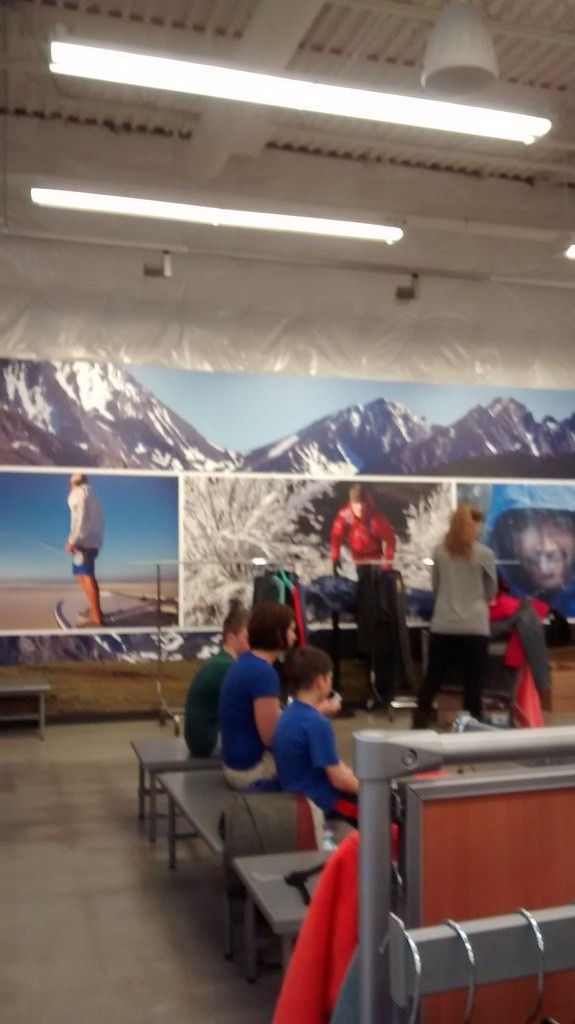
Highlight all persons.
[183,503,497,869]
[332,483,395,592]
[504,508,575,619]
[66,475,107,629]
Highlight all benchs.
[156,769,236,959]
[131,736,191,841]
[232,850,334,984]
[0,675,52,739]
